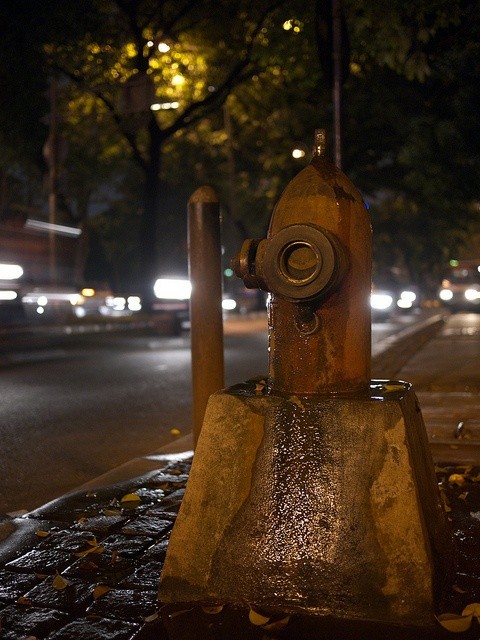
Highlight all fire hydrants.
[228,125,373,396]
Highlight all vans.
[435,265,480,314]
